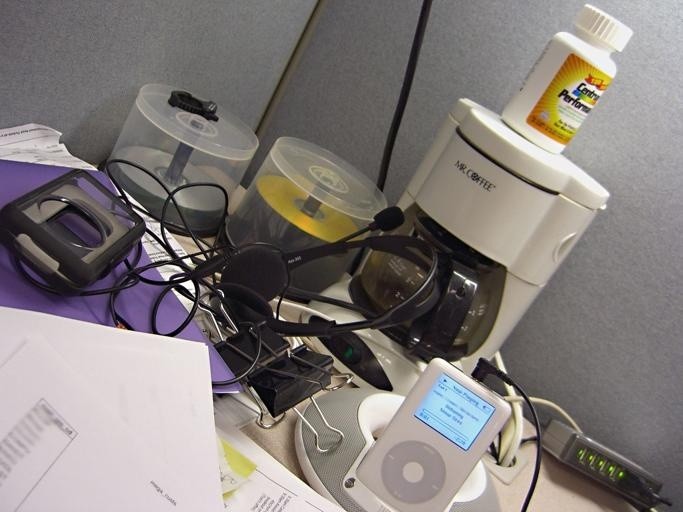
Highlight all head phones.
[212,235,442,336]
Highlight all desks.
[106,165,657,511]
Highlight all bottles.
[502,5,633,153]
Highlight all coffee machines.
[298,95,612,395]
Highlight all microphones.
[333,205,405,241]
